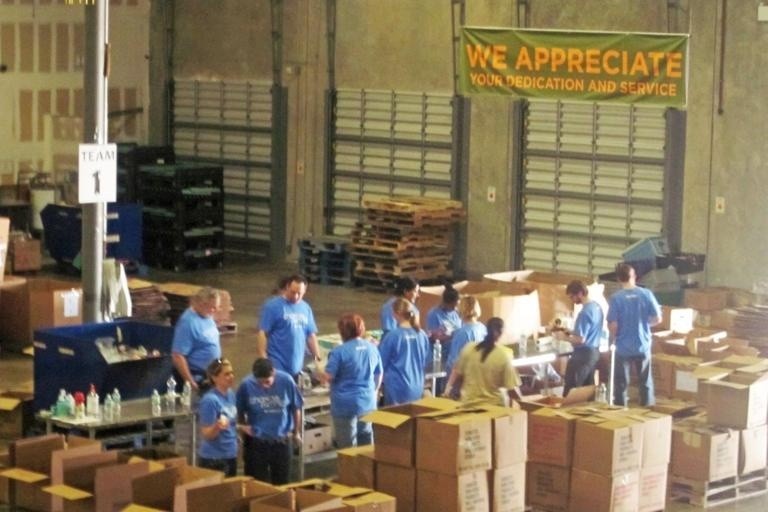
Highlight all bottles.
[152,387,162,416]
[85,383,100,419]
[57,385,85,421]
[433,339,442,361]
[166,374,177,406]
[183,382,191,406]
[598,382,607,402]
[112,388,121,415]
[105,392,113,422]
[519,330,527,348]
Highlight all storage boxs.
[0,217,83,339]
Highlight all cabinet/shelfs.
[141,161,226,273]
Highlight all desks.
[0,184,30,231]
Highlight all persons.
[427,279,461,341]
[378,275,421,338]
[379,297,434,403]
[172,287,222,393]
[315,312,381,449]
[442,317,524,410]
[257,275,321,375]
[236,358,303,484]
[563,281,604,399]
[194,357,239,477]
[607,263,662,405]
[446,293,486,379]
